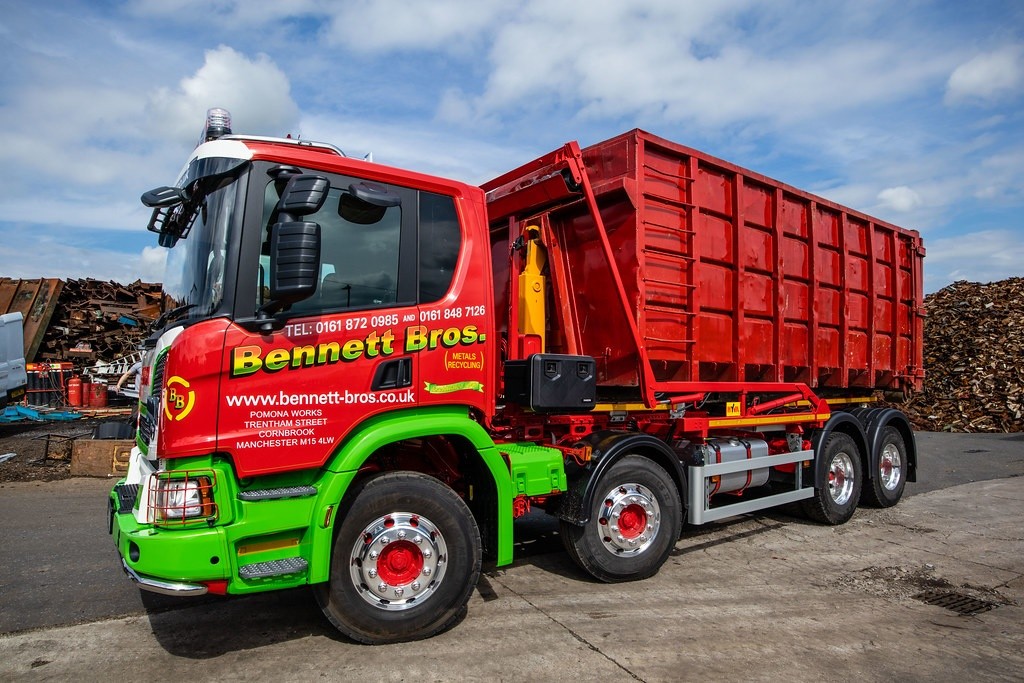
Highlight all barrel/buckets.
[69,379,90,408]
[90,382,106,407]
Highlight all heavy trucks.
[104,106,925,648]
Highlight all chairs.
[321,273,391,304]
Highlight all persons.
[115,362,142,447]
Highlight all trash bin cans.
[97,415,132,439]
